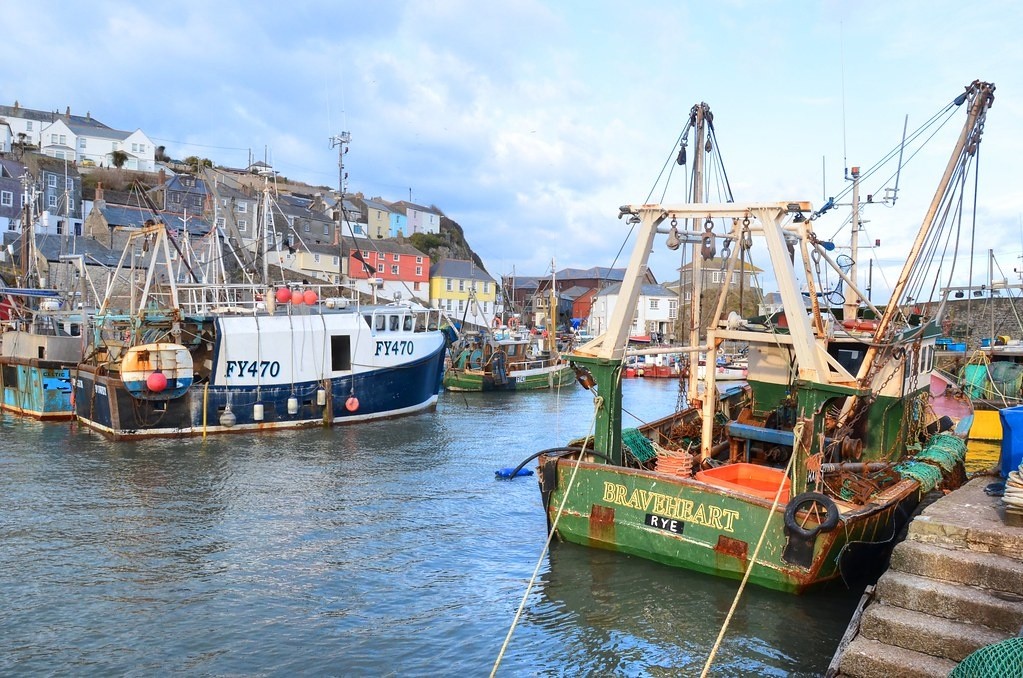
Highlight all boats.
[1,130,751,442]
[535,79,999,598]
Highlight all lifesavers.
[492,318,501,329]
[841,318,880,334]
[508,318,519,328]
[919,415,954,443]
[718,349,722,354]
[783,491,839,538]
[763,405,801,462]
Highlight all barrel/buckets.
[654,448,694,478]
